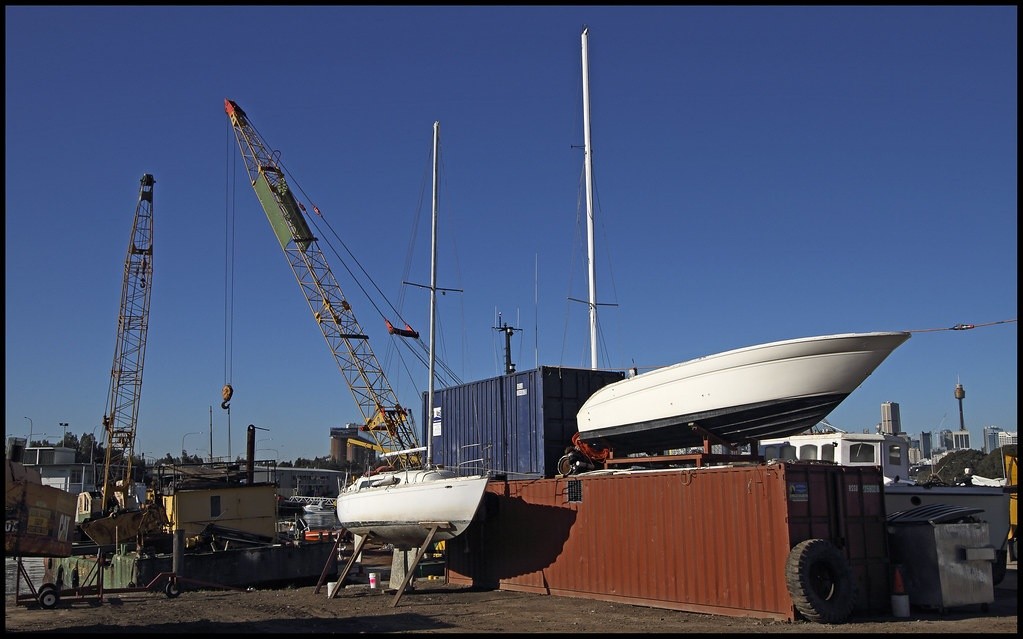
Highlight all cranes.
[81,175,168,546]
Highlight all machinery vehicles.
[220,98,463,472]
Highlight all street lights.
[24,433,46,439]
[182,432,203,449]
[24,417,33,441]
[59,422,68,447]
[256,438,273,450]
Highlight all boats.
[576,331,912,454]
[302,500,335,515]
[305,530,339,541]
[760,433,1010,587]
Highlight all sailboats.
[337,122,488,548]
